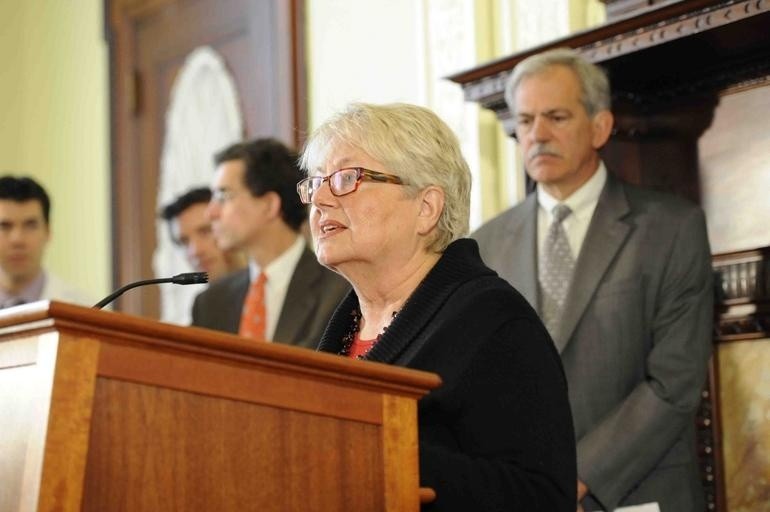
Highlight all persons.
[189,135,353,352]
[470,46,714,512]
[161,187,247,285]
[0,174,79,309]
[296,104,577,512]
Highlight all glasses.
[296,167,409,205]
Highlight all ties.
[538,204,574,333]
[238,274,267,339]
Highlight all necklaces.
[336,306,399,362]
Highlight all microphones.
[90,271,208,310]
